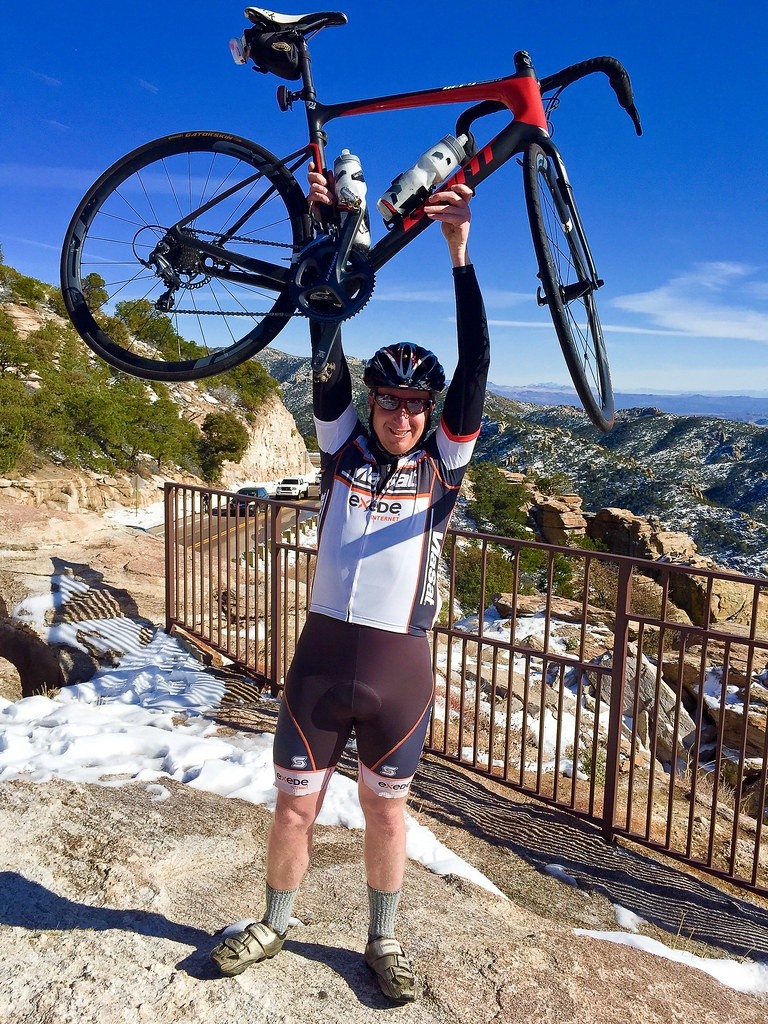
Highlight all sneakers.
[365,938,418,1003]
[210,923,289,977]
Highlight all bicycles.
[60,7,643,431]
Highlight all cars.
[230,487,271,517]
[315,471,322,485]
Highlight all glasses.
[371,391,434,415]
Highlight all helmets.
[362,342,446,392]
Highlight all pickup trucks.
[275,478,309,500]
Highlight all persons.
[204,162,491,1003]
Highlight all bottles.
[377,133,470,224]
[334,150,374,247]
